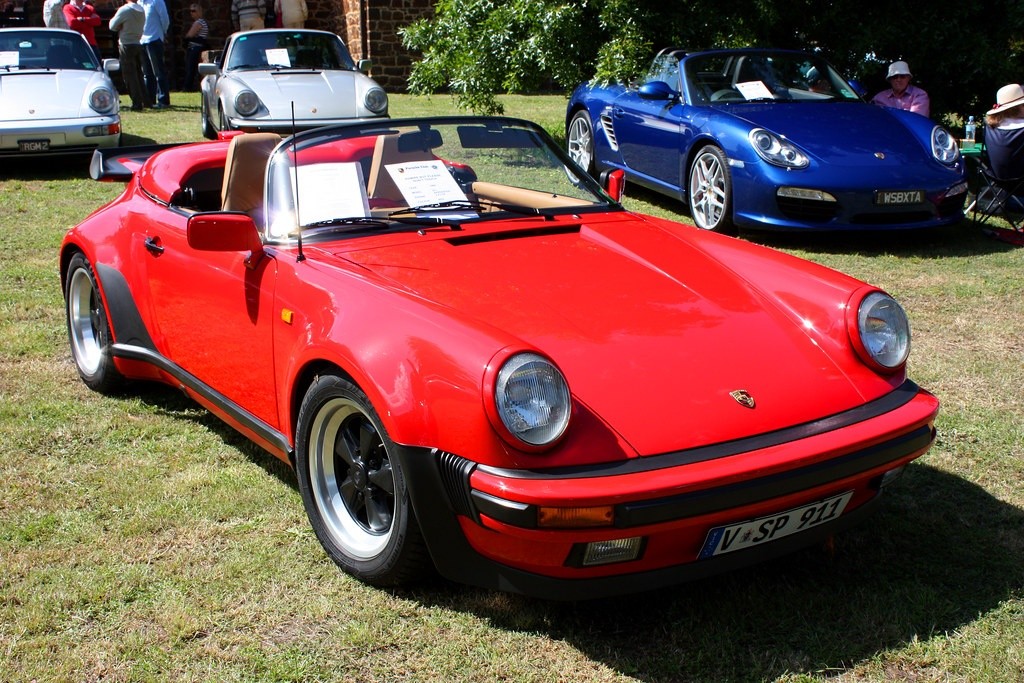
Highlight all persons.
[871,60,930,116]
[803,66,832,93]
[180,2,208,92]
[0,0,102,58]
[108,0,176,112]
[985,84,1024,192]
[232,0,266,30]
[274,0,308,28]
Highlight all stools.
[972,166,1024,237]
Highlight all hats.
[885,61,913,79]
[986,84,1024,115]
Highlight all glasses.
[189,10,196,12]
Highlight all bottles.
[966,115,975,140]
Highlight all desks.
[960,143,987,194]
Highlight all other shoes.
[152,103,169,108]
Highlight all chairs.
[719,56,739,90]
[660,51,698,92]
[221,133,293,231]
[647,47,677,79]
[731,56,774,91]
[294,49,325,68]
[234,46,264,65]
[367,135,433,202]
[46,45,72,68]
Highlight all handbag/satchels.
[276,0,283,28]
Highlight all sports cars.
[564,46,968,241]
[199,29,390,135]
[0,26,121,165]
[59,113,941,593]
